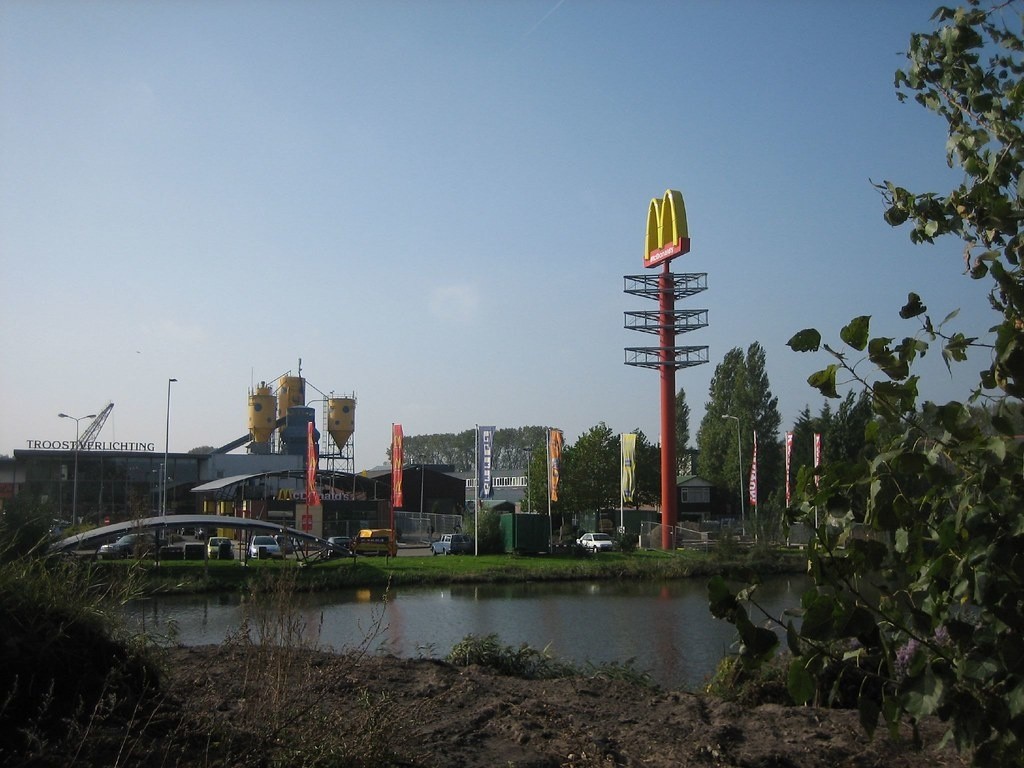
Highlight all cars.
[576,533,613,552]
[194,528,205,540]
[431,534,474,556]
[177,527,194,536]
[327,537,354,558]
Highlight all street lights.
[58,413,97,525]
[419,454,427,517]
[722,414,744,536]
[522,447,533,514]
[162,378,178,515]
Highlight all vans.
[352,528,397,556]
[207,537,232,559]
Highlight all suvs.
[100,534,155,558]
[247,535,280,558]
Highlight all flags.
[478,426,496,497]
[622,432,637,500]
[748,429,823,506]
[392,426,403,506]
[548,427,563,502]
[306,422,320,507]
